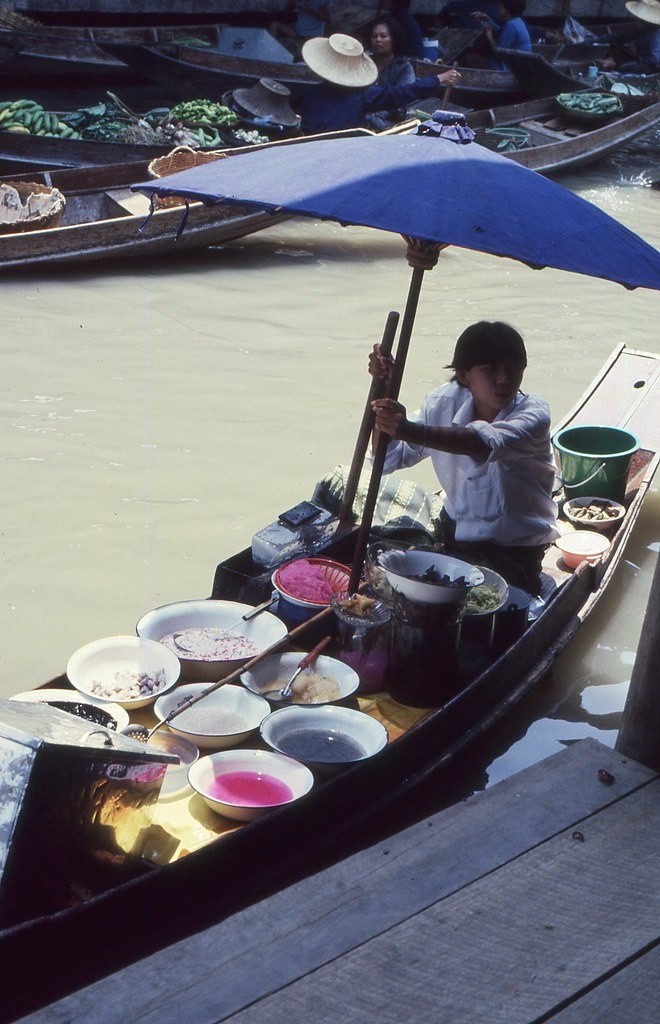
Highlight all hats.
[301,33,379,87]
[624,0,660,25]
[232,78,299,127]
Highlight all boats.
[1,0,660,284]
[0,342,660,1024]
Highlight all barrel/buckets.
[388,615,461,708]
[478,584,533,649]
[551,425,641,501]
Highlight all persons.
[368,321,556,597]
[583,0,660,74]
[268,0,557,128]
[288,34,461,133]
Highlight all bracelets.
[407,422,427,457]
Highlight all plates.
[9,689,130,734]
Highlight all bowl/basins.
[463,564,510,616]
[66,635,181,710]
[187,749,315,822]
[330,591,391,628]
[563,495,626,532]
[366,540,424,601]
[147,729,200,799]
[135,599,289,681]
[240,652,361,710]
[154,682,272,750]
[378,550,485,607]
[259,705,389,775]
[555,530,610,569]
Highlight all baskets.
[553,92,623,125]
[0,181,66,235]
[601,76,660,118]
[147,146,233,208]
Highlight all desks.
[104,189,156,219]
[520,121,582,146]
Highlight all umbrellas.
[131,110,660,596]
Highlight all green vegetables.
[463,583,502,613]
